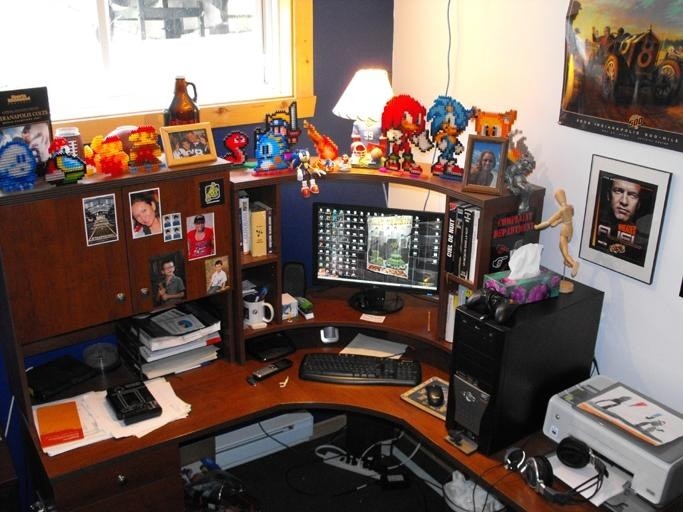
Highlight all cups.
[54,127,83,162]
[243,294,273,324]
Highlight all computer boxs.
[445,276,605,456]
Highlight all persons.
[206,260,227,295]
[187,215,213,259]
[171,131,207,161]
[533,190,582,278]
[164,213,180,242]
[154,260,184,303]
[131,197,160,236]
[606,175,637,224]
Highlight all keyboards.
[298,353,421,386]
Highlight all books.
[130,307,224,379]
[443,284,473,342]
[237,192,274,258]
[443,202,484,284]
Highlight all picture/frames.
[461,134,509,196]
[578,154,673,285]
[160,122,218,168]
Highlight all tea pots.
[169,77,200,126]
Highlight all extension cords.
[323,451,381,480]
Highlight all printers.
[542,375,683,512]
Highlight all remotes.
[252,359,292,379]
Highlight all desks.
[18,326,613,512]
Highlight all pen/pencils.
[248,287,268,302]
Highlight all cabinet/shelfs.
[1,158,242,353]
[228,156,545,364]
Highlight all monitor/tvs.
[320,202,444,315]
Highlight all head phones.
[522,436,609,506]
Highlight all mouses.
[425,384,444,406]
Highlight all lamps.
[331,68,396,142]
